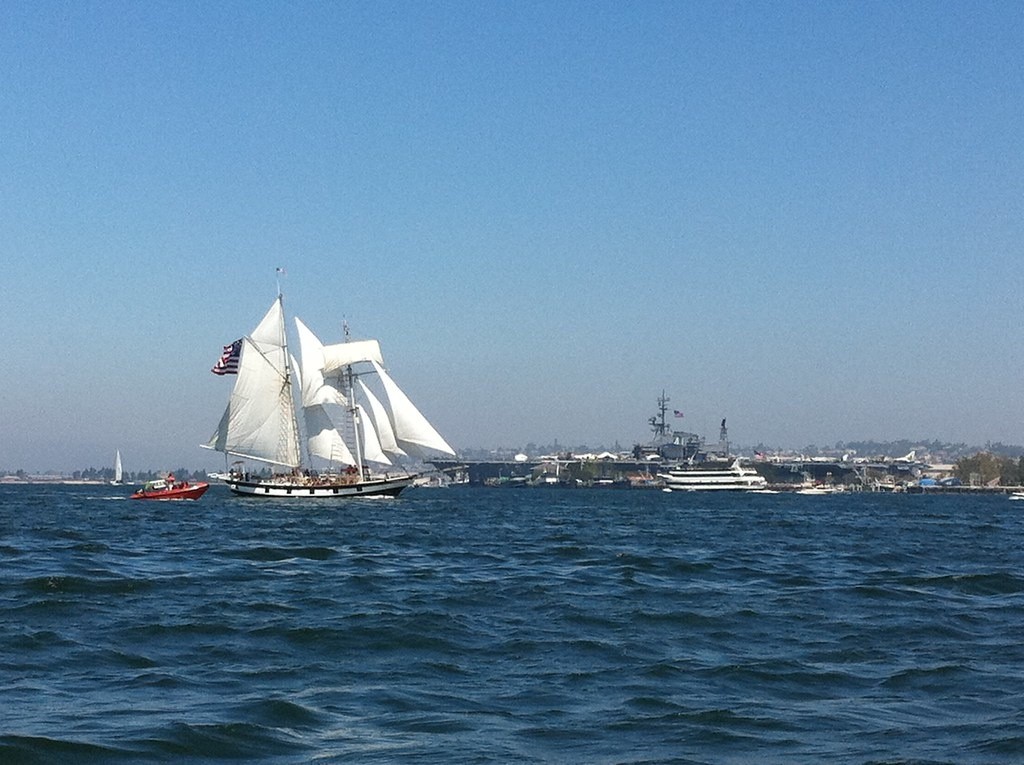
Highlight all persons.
[229,463,374,485]
[166,471,175,490]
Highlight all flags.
[211,338,243,376]
[674,410,684,417]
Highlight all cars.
[919,479,938,485]
[935,477,963,487]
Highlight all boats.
[129,470,210,501]
[144,478,167,490]
[796,488,833,495]
[657,466,769,491]
[1008,491,1024,501]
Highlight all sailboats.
[199,267,470,502]
[109,448,124,488]
